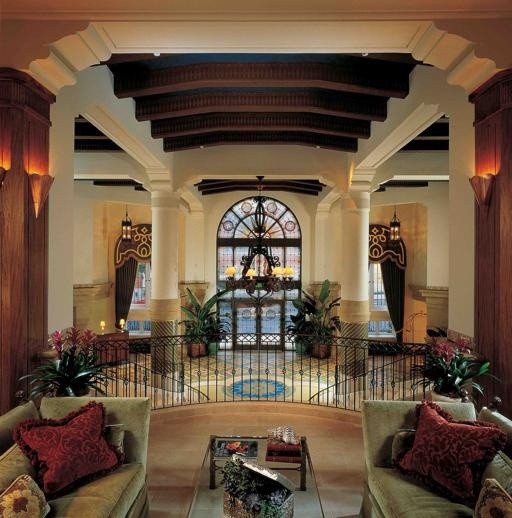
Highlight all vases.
[431,390,469,402]
[81,389,92,397]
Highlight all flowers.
[17,327,110,397]
[410,335,491,398]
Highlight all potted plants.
[285,321,312,355]
[205,312,230,355]
[292,278,341,359]
[178,286,233,358]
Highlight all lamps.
[224,175,295,306]
[390,205,401,242]
[121,203,133,241]
[119,319,126,332]
[99,320,105,335]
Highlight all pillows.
[481,449,512,497]
[12,400,123,501]
[0,442,37,495]
[391,427,417,469]
[392,400,508,511]
[105,424,126,463]
[0,474,52,517]
[472,477,512,518]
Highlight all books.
[213,440,258,461]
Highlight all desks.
[94,328,129,367]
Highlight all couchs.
[358,400,512,518]
[0,397,151,517]
